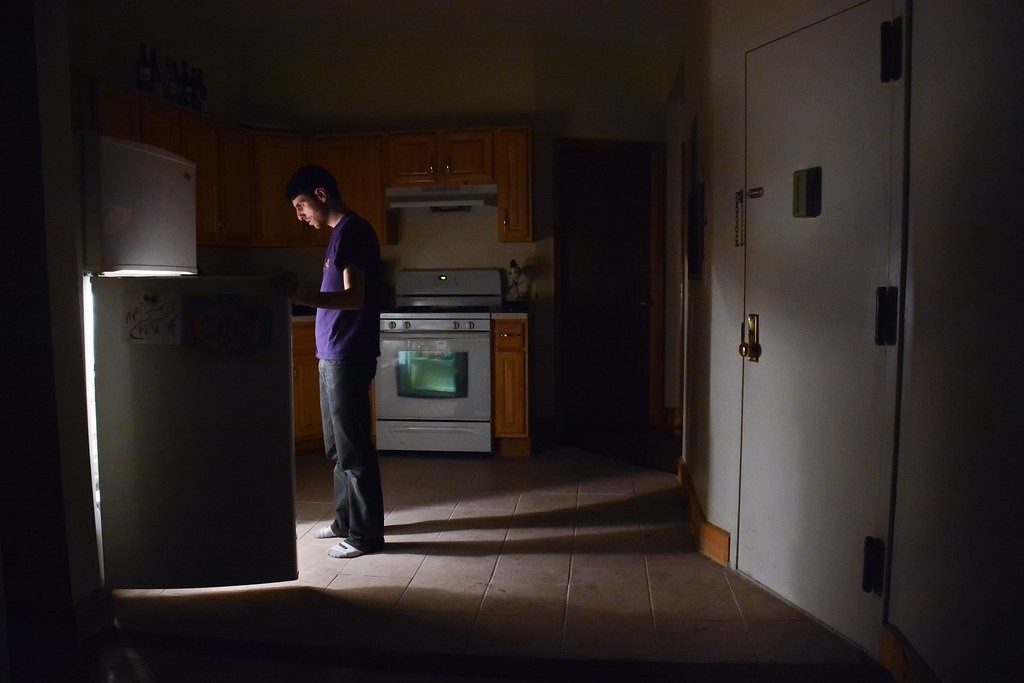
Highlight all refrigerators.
[50,133,300,599]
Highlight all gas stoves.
[380,263,505,319]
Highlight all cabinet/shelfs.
[289,308,530,458]
[69,76,536,251]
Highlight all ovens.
[376,319,490,423]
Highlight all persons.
[267,167,388,557]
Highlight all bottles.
[507,259,520,300]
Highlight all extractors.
[387,181,498,217]
